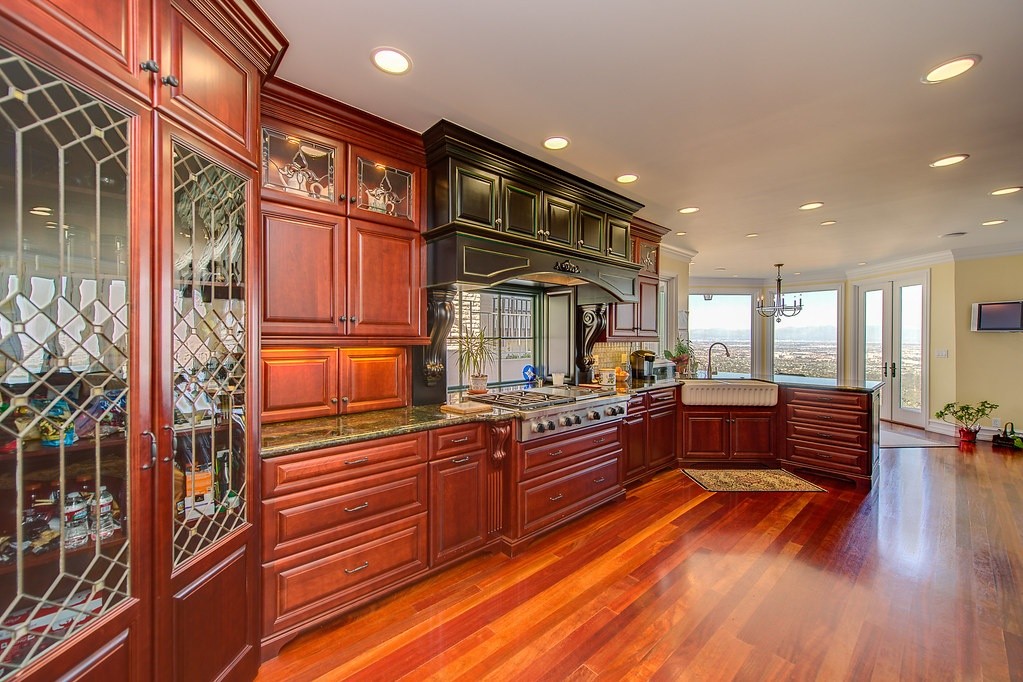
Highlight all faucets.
[707,342,730,379]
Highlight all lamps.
[756,264,805,323]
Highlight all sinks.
[679,380,723,405]
[724,380,779,406]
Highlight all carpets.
[680,468,828,493]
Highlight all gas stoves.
[466,390,577,411]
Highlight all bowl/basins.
[616,376,629,382]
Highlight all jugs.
[365,189,395,215]
[309,182,334,203]
[279,165,309,197]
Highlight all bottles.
[63,493,89,550]
[90,487,114,540]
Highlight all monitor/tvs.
[971,301,1023,333]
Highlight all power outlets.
[593,355,599,367]
[621,354,628,364]
[991,418,1002,428]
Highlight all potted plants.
[664,337,695,372]
[935,400,999,442]
[448,323,500,395]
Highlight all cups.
[599,369,616,386]
[551,372,565,386]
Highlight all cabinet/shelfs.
[577,176,645,262]
[647,387,679,478]
[499,143,582,250]
[261,345,413,424]
[0,0,290,682]
[622,391,647,489]
[780,387,882,490]
[422,118,501,231]
[595,216,672,343]
[502,418,627,559]
[0,418,244,616]
[259,76,428,232]
[260,431,430,663]
[260,200,432,346]
[428,422,502,578]
[682,411,778,470]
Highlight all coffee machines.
[630,350,657,380]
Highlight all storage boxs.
[184,492,215,520]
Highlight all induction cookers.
[522,388,617,401]
